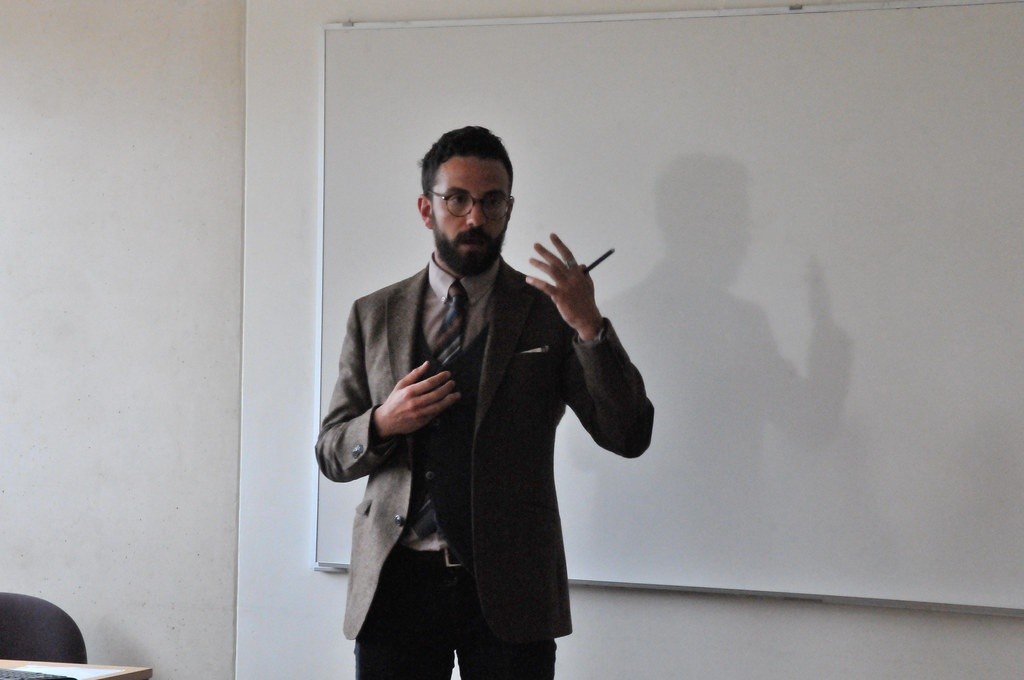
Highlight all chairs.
[0,592,87,664]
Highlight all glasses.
[425,187,513,220]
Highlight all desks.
[0,660,153,680]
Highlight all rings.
[565,258,577,268]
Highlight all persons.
[314,127,654,680]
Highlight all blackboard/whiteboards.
[309,1,1024,618]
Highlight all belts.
[387,544,467,571]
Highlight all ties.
[404,278,468,540]
